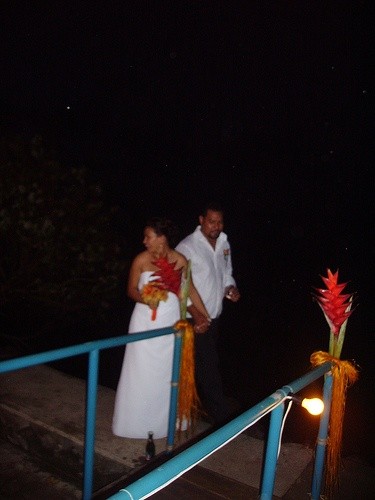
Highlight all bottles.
[145,431,156,462]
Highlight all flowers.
[316,268,356,357]
[142,253,195,329]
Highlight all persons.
[110,221,212,440]
[175,203,241,429]
